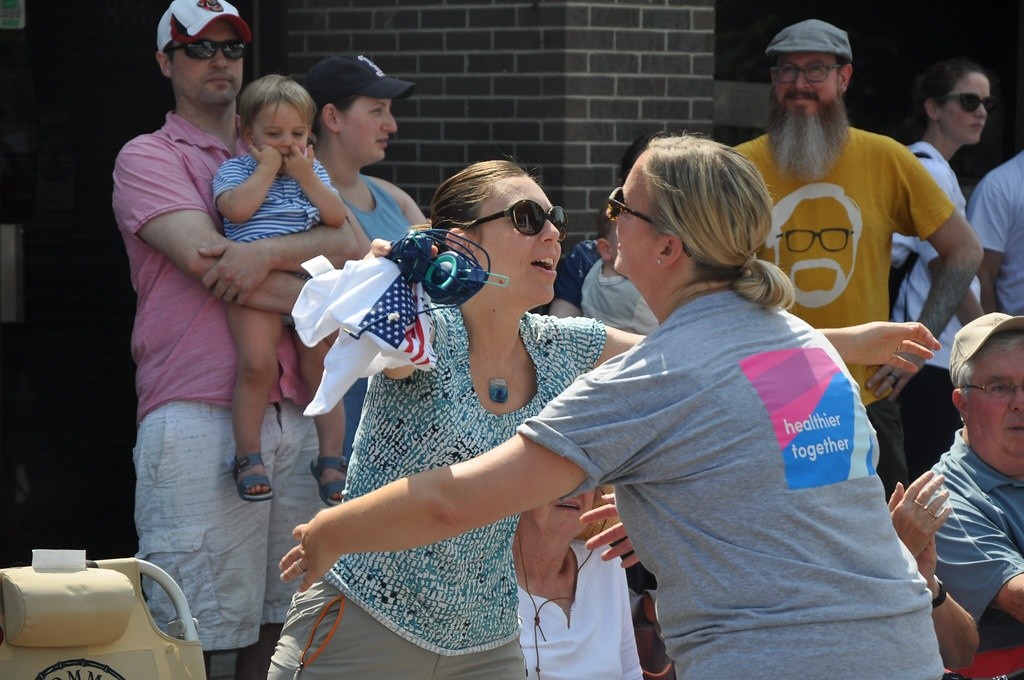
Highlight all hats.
[304,55,417,105]
[950,312,1024,388]
[575,485,622,544]
[157,0,252,52]
[766,18,852,61]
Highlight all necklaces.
[462,314,518,403]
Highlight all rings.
[895,344,901,352]
[296,559,307,574]
[926,509,935,517]
[886,372,899,387]
[914,499,925,507]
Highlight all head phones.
[385,229,510,305]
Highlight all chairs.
[0,557,206,680]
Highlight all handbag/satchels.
[633,590,676,680]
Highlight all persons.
[113,0,1024,680]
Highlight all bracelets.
[932,574,947,609]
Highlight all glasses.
[605,187,692,258]
[941,93,998,113]
[466,200,568,241]
[960,379,1024,400]
[168,38,249,60]
[772,61,842,83]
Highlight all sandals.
[310,456,349,506]
[230,452,275,502]
